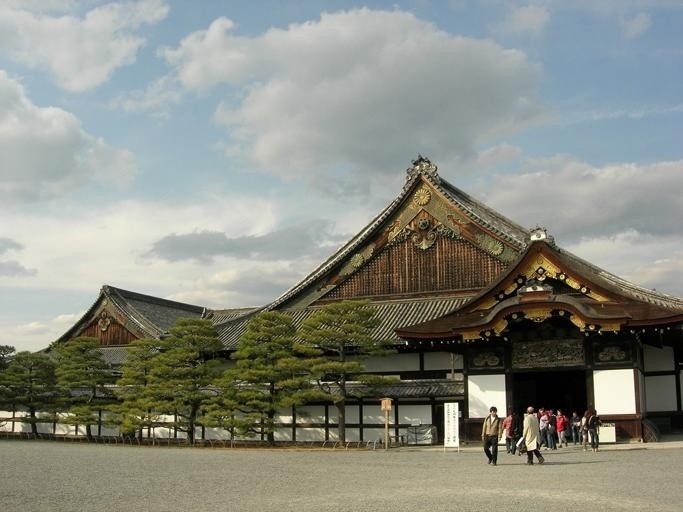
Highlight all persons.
[537,407,602,453]
[501,407,522,456]
[480,407,503,466]
[522,406,544,466]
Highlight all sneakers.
[488,459,497,466]
[558,442,567,448]
[538,459,544,463]
[543,444,557,451]
[592,448,600,452]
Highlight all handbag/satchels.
[516,438,525,448]
